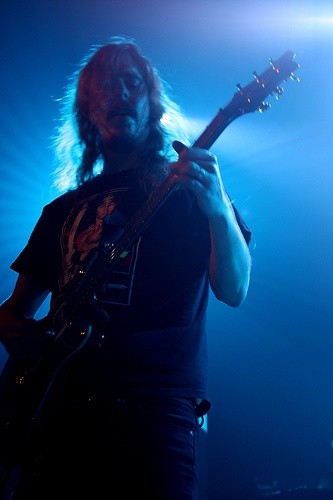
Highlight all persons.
[0,37,299,497]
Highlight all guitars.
[0,48,300,467]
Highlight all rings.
[196,167,207,180]
